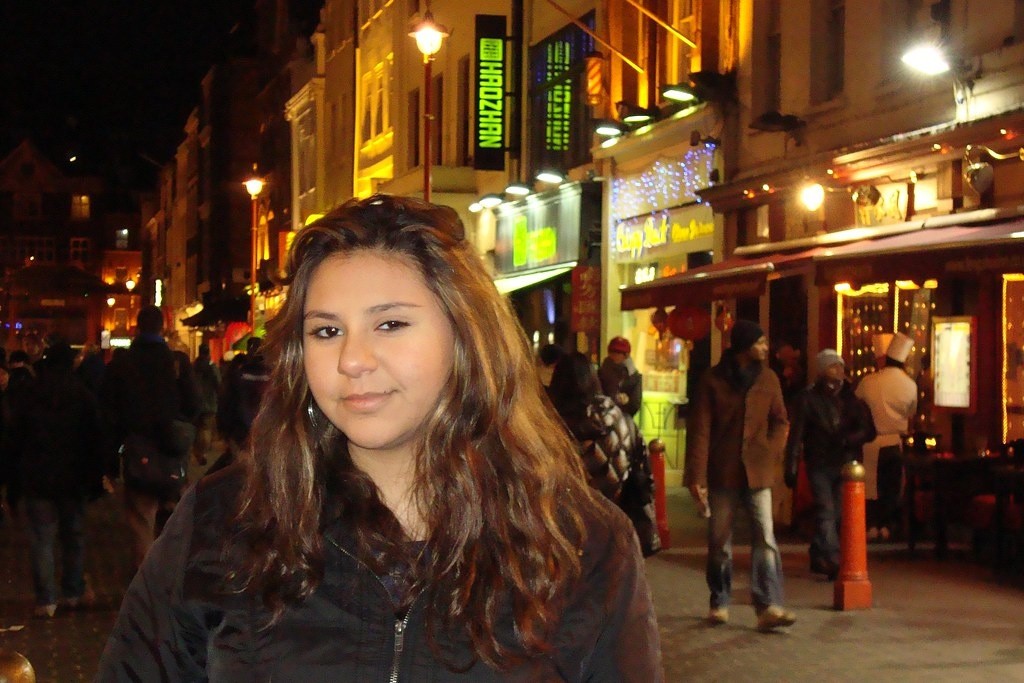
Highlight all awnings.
[493,262,577,297]
[618,205,1024,312]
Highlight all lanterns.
[652,308,669,342]
[669,305,709,350]
[208,320,252,367]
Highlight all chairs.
[901,432,942,551]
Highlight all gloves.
[783,469,798,488]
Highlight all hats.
[608,337,631,354]
[886,331,915,362]
[816,349,845,371]
[872,333,894,360]
[730,319,765,354]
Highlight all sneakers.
[709,606,728,624]
[757,606,797,633]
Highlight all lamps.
[504,183,534,197]
[615,100,661,124]
[801,182,857,211]
[478,194,503,208]
[590,118,626,137]
[687,71,733,103]
[747,110,800,133]
[535,168,565,185]
[900,42,979,89]
[662,82,699,107]
[964,143,1024,190]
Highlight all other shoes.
[34,601,57,618]
[63,597,78,606]
[642,544,661,558]
[809,552,839,575]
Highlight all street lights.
[106,295,116,351]
[124,275,137,344]
[242,161,270,341]
[407,11,454,202]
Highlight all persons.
[92,194,664,683]
[854,333,918,543]
[105,305,203,581]
[538,336,662,562]
[785,349,878,581]
[683,320,796,632]
[6,343,107,621]
[0,332,273,523]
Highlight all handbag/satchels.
[123,435,186,497]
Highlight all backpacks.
[239,363,274,393]
[620,455,652,513]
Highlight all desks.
[924,454,1024,571]
